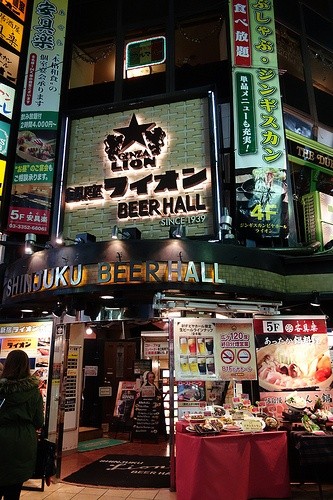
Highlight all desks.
[176,431,288,500]
[281,427,333,500]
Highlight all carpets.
[77,437,130,453]
[62,453,176,487]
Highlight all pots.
[235,178,287,204]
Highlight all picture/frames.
[99,387,112,397]
[85,366,98,376]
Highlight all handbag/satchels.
[29,425,57,480]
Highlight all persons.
[145,371,161,399]
[0,350,44,500]
[295,125,302,134]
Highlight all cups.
[180,337,215,376]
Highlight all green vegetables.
[302,415,319,431]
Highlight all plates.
[184,404,266,433]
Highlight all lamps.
[56,232,83,245]
[66,307,76,318]
[24,233,51,254]
[112,225,130,239]
[306,241,321,255]
[51,302,66,317]
[173,224,182,237]
[220,207,235,238]
